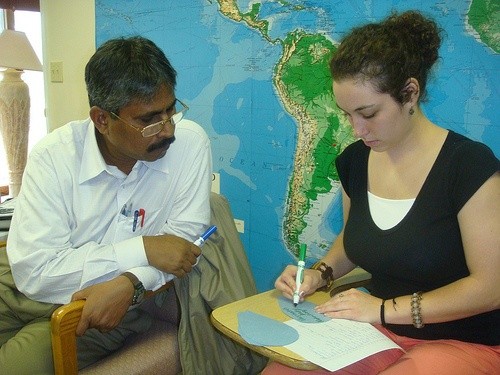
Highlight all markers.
[195,226,217,247]
[293,243,307,307]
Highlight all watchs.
[121,272,146,306]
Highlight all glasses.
[104,96,189,138]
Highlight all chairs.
[51,190,269,375]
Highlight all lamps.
[0,29,44,196]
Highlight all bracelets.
[379,297,388,328]
[409,290,426,329]
[308,261,335,291]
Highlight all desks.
[210,267,372,371]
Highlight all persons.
[259,6,500,375]
[0,34,214,374]
